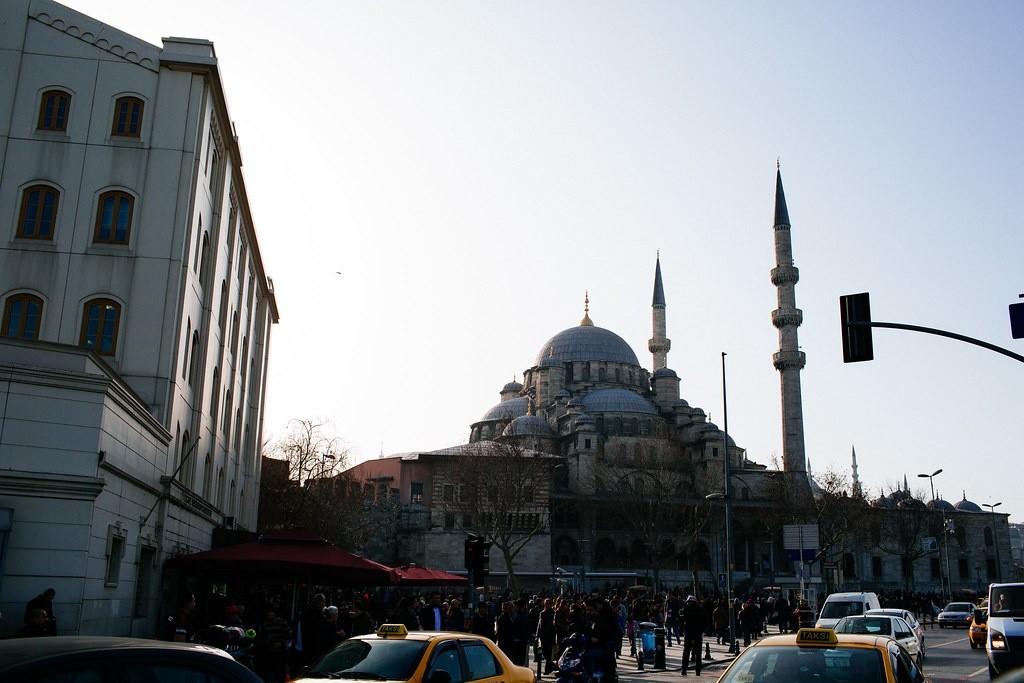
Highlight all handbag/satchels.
[723,625,731,642]
[551,645,559,662]
[534,638,544,662]
[772,611,778,619]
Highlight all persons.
[682,594,706,676]
[891,588,925,618]
[158,585,814,683]
[10,588,58,639]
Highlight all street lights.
[982,502,1003,583]
[943,519,955,601]
[706,494,735,652]
[316,454,335,535]
[917,468,946,605]
[643,544,653,595]
[542,465,563,592]
[578,540,592,592]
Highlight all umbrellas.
[175,526,402,620]
[393,562,469,596]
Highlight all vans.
[974,583,1024,680]
[815,592,882,632]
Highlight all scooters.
[553,631,619,683]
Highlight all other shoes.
[722,642,726,645]
[696,672,700,676]
[678,641,680,644]
[616,651,621,659]
[682,671,687,675]
[544,667,552,674]
[752,634,762,639]
[780,629,787,633]
[717,638,720,644]
[629,651,636,657]
[668,644,672,647]
[744,641,751,647]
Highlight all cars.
[823,615,923,673]
[0,638,262,683]
[286,624,535,683]
[967,596,988,650]
[862,609,925,656]
[937,602,977,628]
[715,629,933,683]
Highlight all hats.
[478,602,488,607]
[685,595,697,602]
[742,603,748,609]
[746,599,753,603]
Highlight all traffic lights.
[465,539,475,561]
[479,537,489,577]
